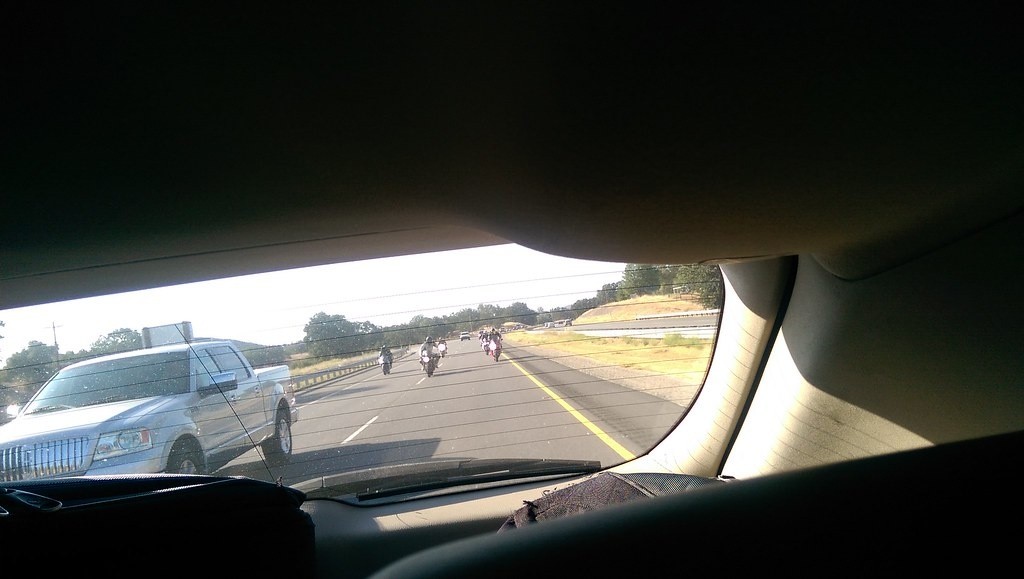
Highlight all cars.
[507,324,524,332]
[679,287,688,295]
[499,327,507,333]
[548,321,556,328]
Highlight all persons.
[481,330,489,340]
[489,328,502,342]
[478,329,483,339]
[379,345,394,368]
[419,336,440,371]
[438,336,448,350]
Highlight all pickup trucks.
[0,340,299,491]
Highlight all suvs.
[565,319,572,327]
[460,332,471,341]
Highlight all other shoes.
[435,363,438,368]
[421,366,425,371]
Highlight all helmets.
[426,336,433,342]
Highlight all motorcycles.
[378,353,394,375]
[438,343,449,359]
[420,350,439,377]
[482,338,492,355]
[489,338,503,362]
